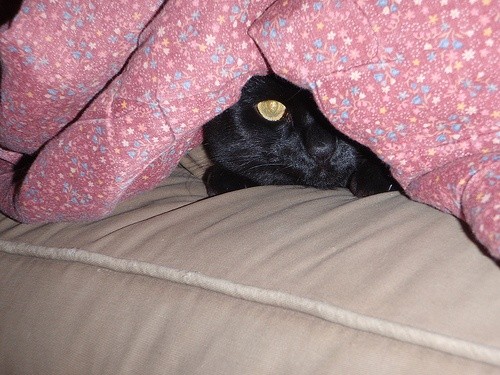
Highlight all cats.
[201,75,390,199]
